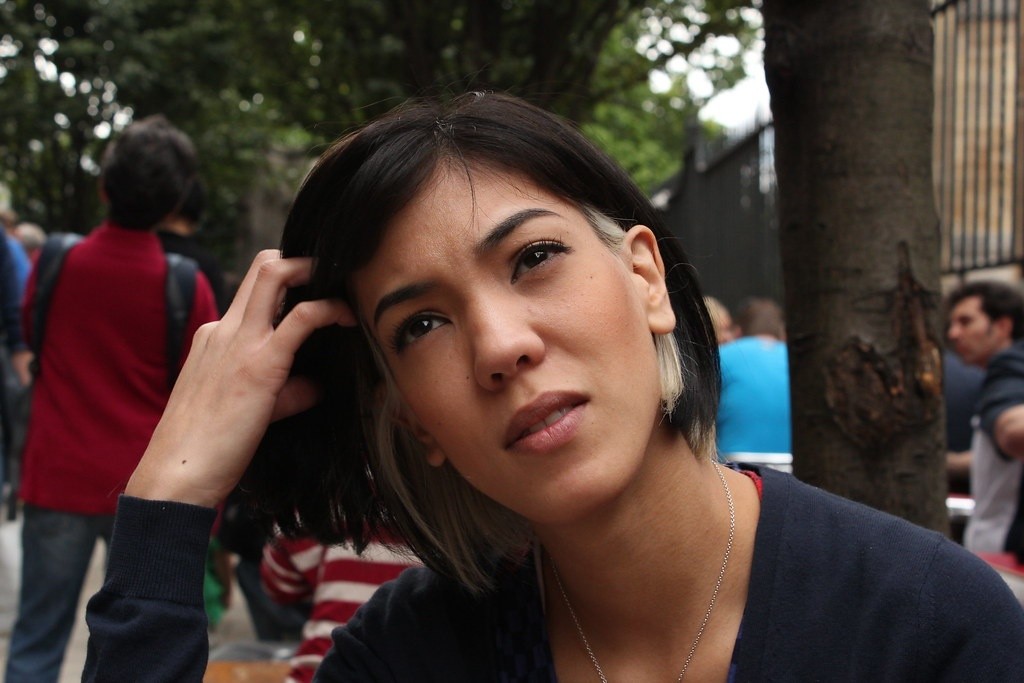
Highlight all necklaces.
[548,459,735,683]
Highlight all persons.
[700,296,791,456]
[941,280,1023,576]
[78,92,1024,683]
[228,508,428,683]
[0,113,225,683]
